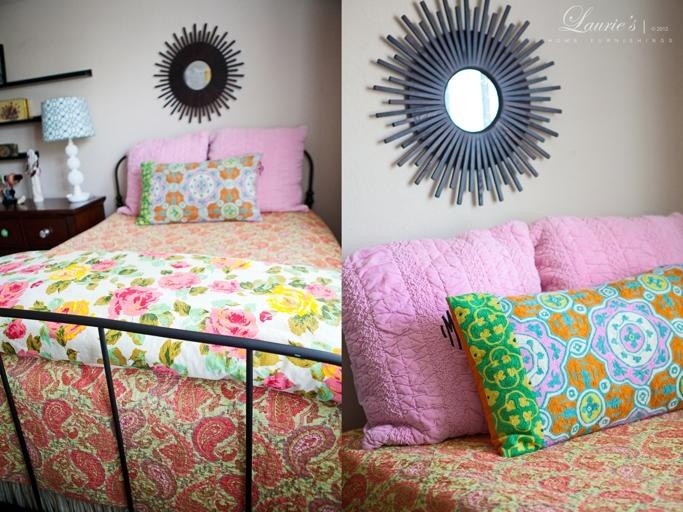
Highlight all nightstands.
[0,197,106,255]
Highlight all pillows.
[117,133,210,216]
[444,262,683,459]
[530,211,683,289]
[339,220,542,451]
[136,153,264,226]
[208,126,310,213]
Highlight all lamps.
[40,96,94,203]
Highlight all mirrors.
[373,1,563,207]
[152,22,244,126]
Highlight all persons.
[1,173,26,206]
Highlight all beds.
[340,410,682,512]
[0,148,341,512]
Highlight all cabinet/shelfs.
[0,45,92,160]
